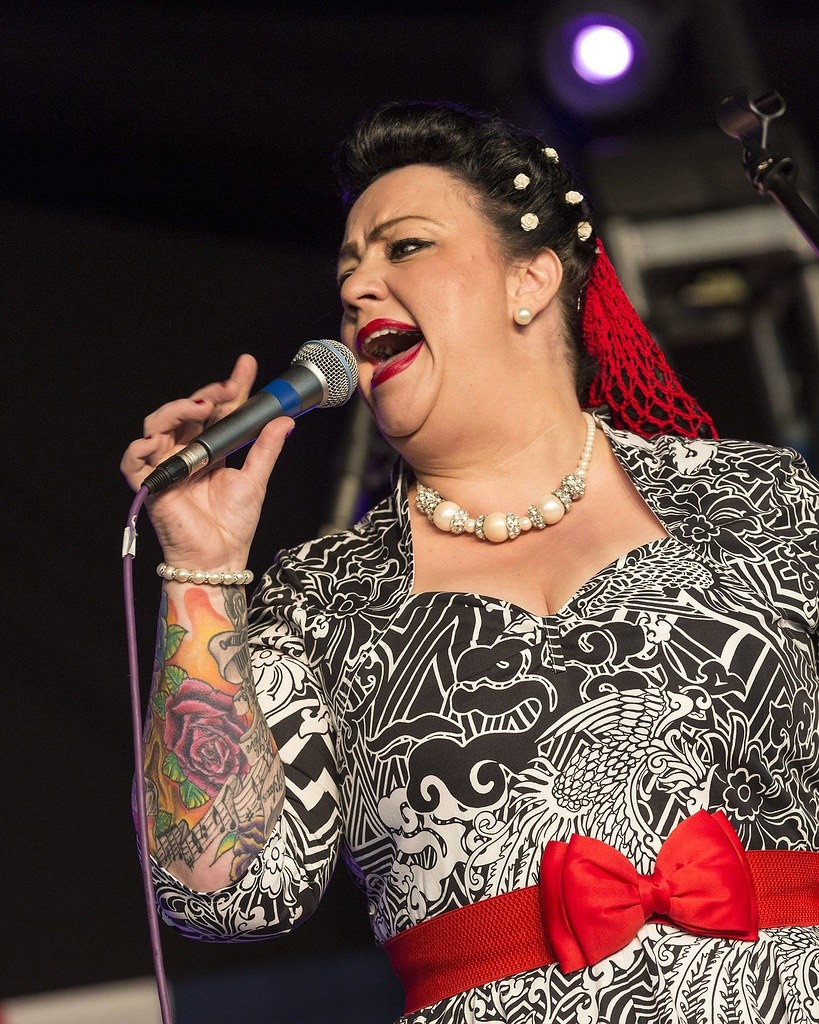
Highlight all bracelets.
[156,563,254,587]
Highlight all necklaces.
[418,411,599,546]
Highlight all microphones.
[141,340,359,494]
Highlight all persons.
[119,88,819,1024]
